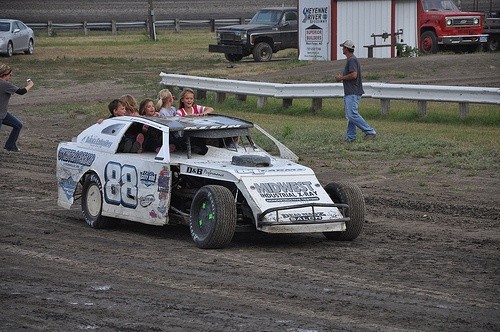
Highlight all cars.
[482,10,500,53]
[56,112,366,250]
[0,19,35,57]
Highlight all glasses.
[168,96,175,99]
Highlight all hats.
[340,40,354,49]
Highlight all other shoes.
[365,134,375,141]
[5,146,20,152]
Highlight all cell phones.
[27,79,30,84]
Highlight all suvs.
[208,6,299,63]
[417,0,490,55]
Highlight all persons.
[335,40,378,143]
[94,86,241,155]
[0,64,34,152]
